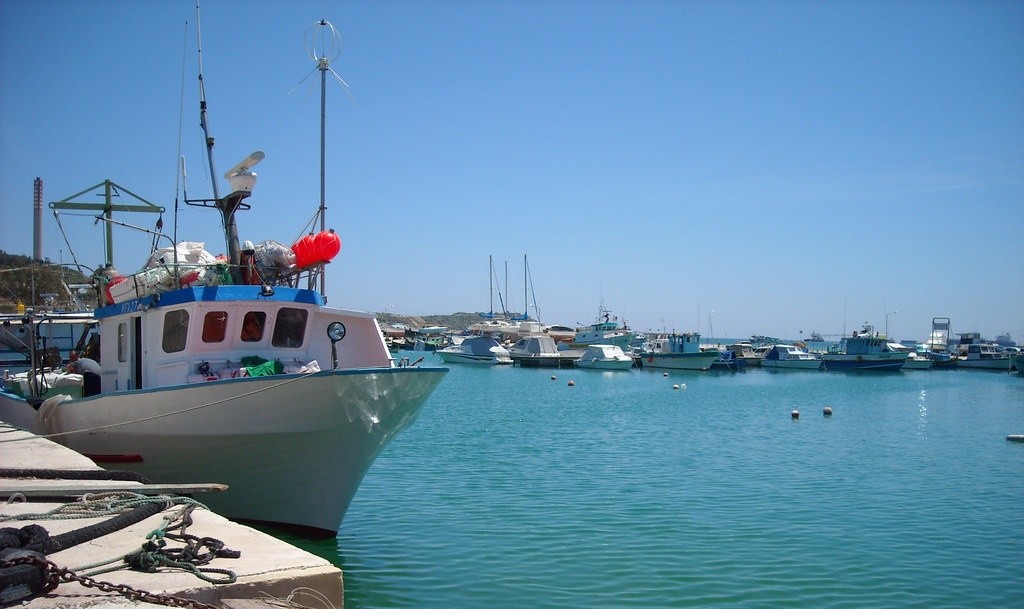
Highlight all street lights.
[706,309,715,348]
[886,311,898,337]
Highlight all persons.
[66,358,101,397]
[68,351,80,364]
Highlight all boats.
[0,253,1024,378]
[0,0,451,542]
[573,343,635,372]
